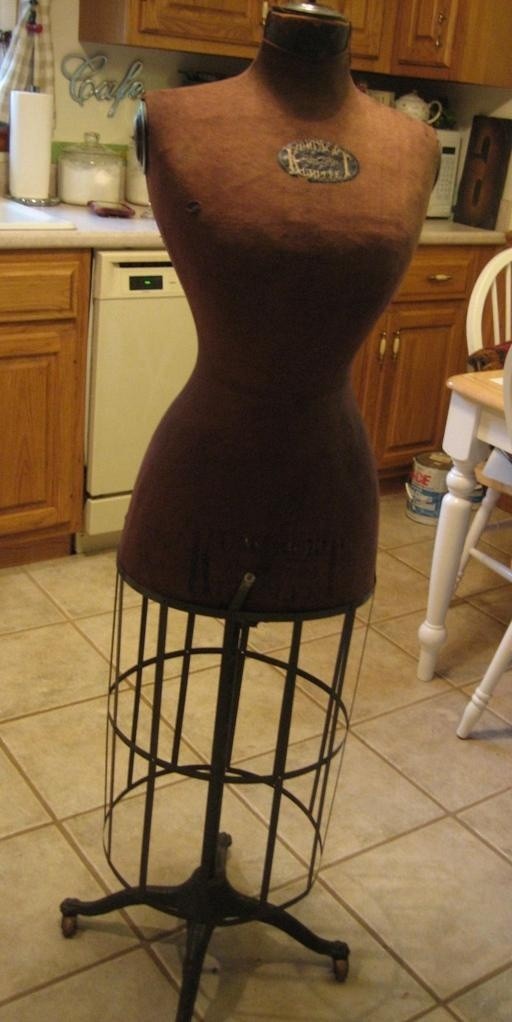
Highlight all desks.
[417,371,505,685]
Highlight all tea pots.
[390,89,443,125]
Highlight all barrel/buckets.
[404,450,454,526]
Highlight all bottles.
[57,132,125,206]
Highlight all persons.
[115,1,440,622]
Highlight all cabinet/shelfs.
[79,0,512,88]
[0,246,92,568]
[351,245,506,496]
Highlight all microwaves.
[426,129,462,219]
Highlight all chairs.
[456,344,512,740]
[454,247,512,596]
